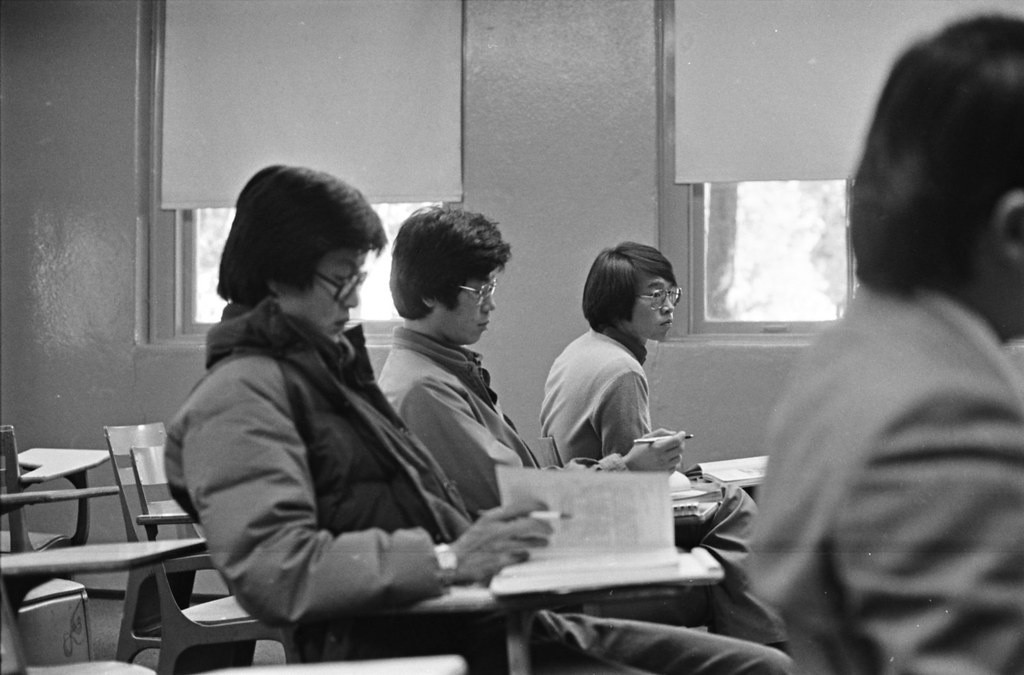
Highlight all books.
[682,547,723,582]
[492,464,693,597]
[670,471,722,522]
[687,455,769,489]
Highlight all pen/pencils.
[633,434,694,444]
[477,508,573,520]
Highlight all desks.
[0,538,210,575]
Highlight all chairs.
[0,421,511,675]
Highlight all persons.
[165,165,791,675]
[380,207,717,628]
[541,244,682,465]
[748,15,1023,675]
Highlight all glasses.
[309,271,366,300]
[460,277,497,303]
[639,287,681,309]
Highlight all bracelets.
[433,542,458,587]
